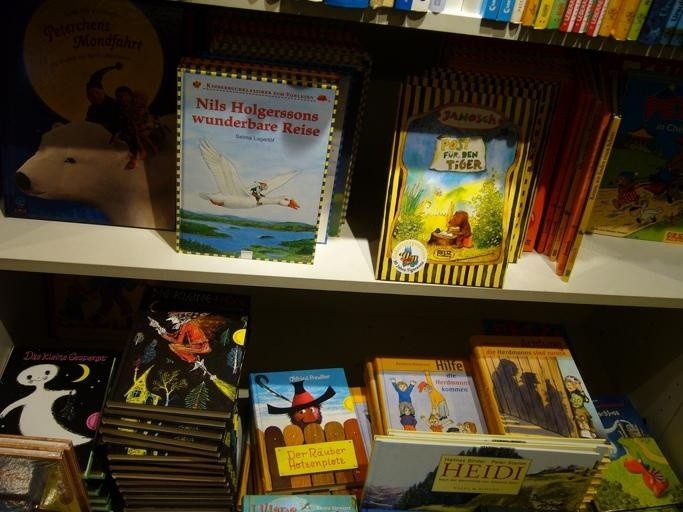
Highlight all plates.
[0,0,683,510]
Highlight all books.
[0,284,683,511]
[376,48,620,283]
[615,65,681,161]
[298,0,683,47]
[178,31,371,263]
